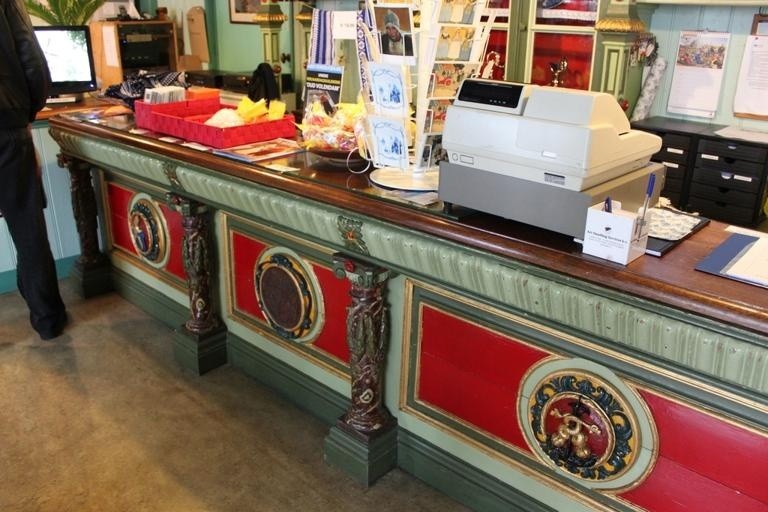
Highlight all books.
[645,207,710,257]
[212,138,304,163]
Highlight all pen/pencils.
[606,174,655,216]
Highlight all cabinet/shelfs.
[630,115,768,229]
[89,18,180,93]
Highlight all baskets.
[134,91,296,149]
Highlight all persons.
[0,0,72,340]
[379,10,412,55]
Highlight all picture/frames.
[372,2,417,60]
[228,0,261,25]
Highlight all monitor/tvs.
[31,25,98,103]
